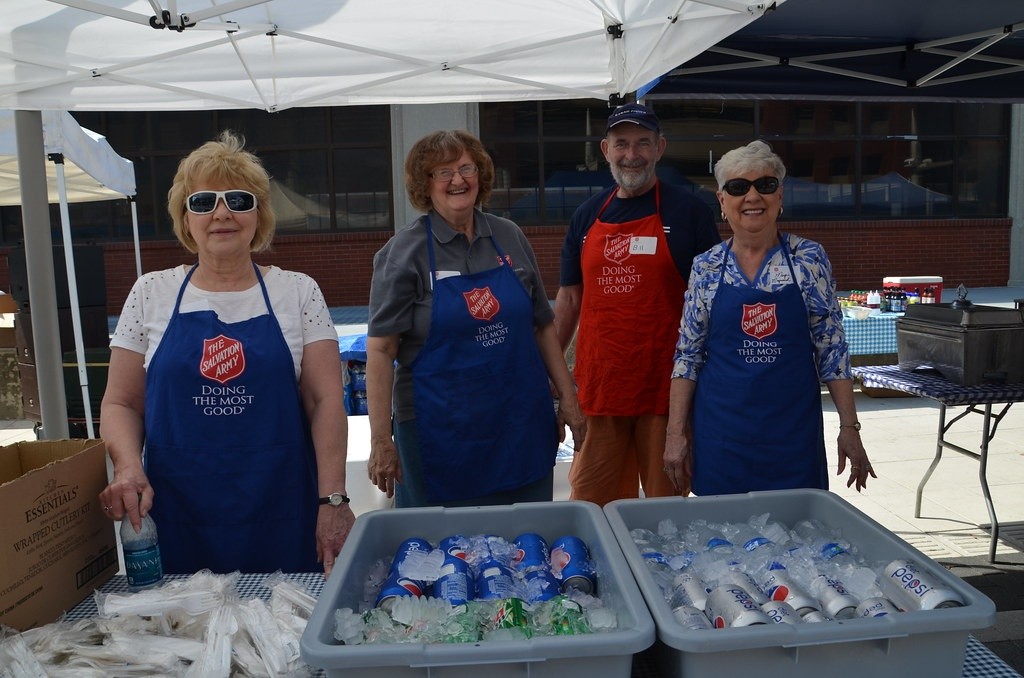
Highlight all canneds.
[668,558,970,630]
[359,534,597,643]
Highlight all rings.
[866,465,871,471]
[104,505,112,512]
[852,465,860,470]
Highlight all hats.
[604,102,658,136]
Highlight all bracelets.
[840,422,861,431]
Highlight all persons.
[552,104,722,510]
[665,145,877,498]
[100,128,357,581]
[366,128,588,508]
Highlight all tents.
[0,110,142,438]
[831,171,951,203]
[778,175,856,220]
[1,0,783,112]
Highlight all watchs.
[320,490,351,508]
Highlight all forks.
[0,570,319,678]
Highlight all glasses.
[719,175,780,197]
[429,163,480,182]
[184,189,258,215]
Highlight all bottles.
[927,288,935,303]
[874,290,880,308]
[906,292,915,304]
[861,292,866,307]
[120,493,164,593]
[858,291,861,306]
[850,290,854,301]
[867,290,875,308]
[879,293,886,311]
[883,286,901,312]
[901,287,907,312]
[837,297,858,308]
[631,518,854,592]
[915,288,920,304]
[854,291,858,301]
[921,288,928,304]
[342,364,367,416]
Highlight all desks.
[58,572,1020,678]
[851,364,1024,562]
[843,315,898,355]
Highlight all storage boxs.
[300,500,657,678]
[883,276,943,303]
[603,488,996,678]
[0,438,119,636]
[860,379,916,398]
